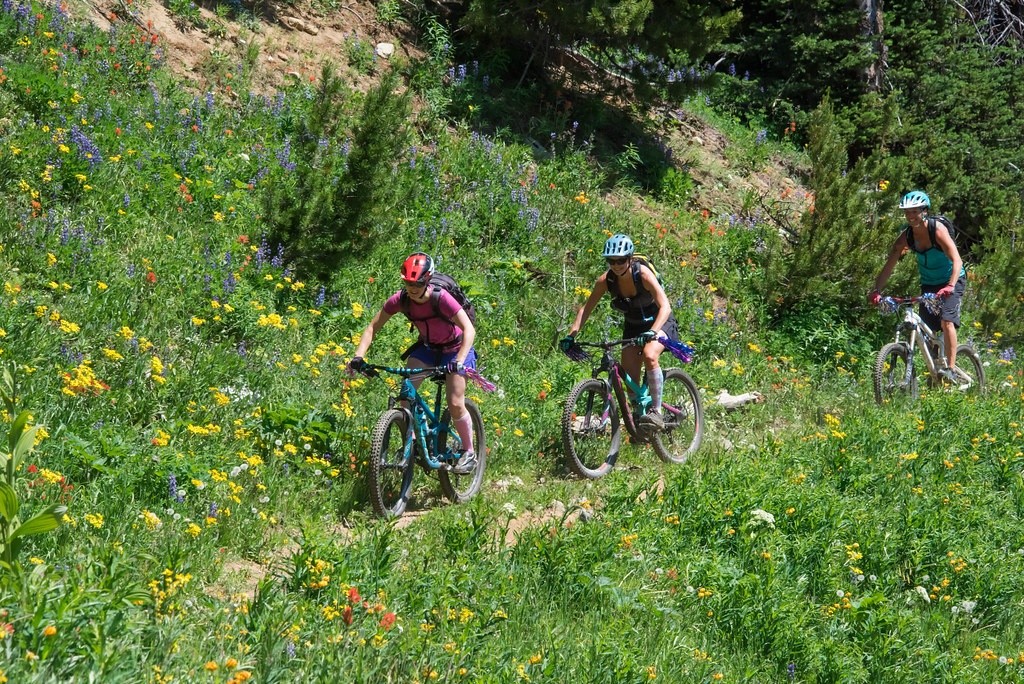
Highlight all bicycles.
[368,363,486,521]
[873,293,986,406]
[561,337,704,480]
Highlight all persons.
[557,232,679,444]
[868,191,966,386]
[347,252,477,474]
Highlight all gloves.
[446,360,462,375]
[351,357,364,373]
[869,291,881,306]
[559,335,575,353]
[936,285,955,297]
[634,330,657,348]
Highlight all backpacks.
[399,271,476,326]
[905,216,956,252]
[607,252,664,301]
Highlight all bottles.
[419,408,430,437]
[631,399,641,428]
[427,424,435,457]
[924,337,936,359]
[934,343,940,359]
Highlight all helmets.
[602,234,634,256]
[401,253,434,282]
[899,191,930,209]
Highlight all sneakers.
[453,452,478,473]
[640,406,665,428]
[937,367,958,382]
[629,431,649,443]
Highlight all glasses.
[605,257,627,265]
[403,280,426,288]
